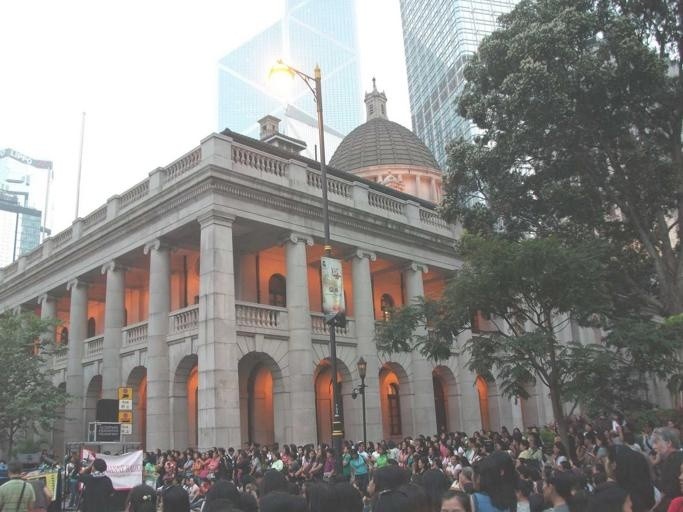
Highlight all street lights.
[351,355,368,454]
[269,57,345,478]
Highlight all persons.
[0,411,683,512]
